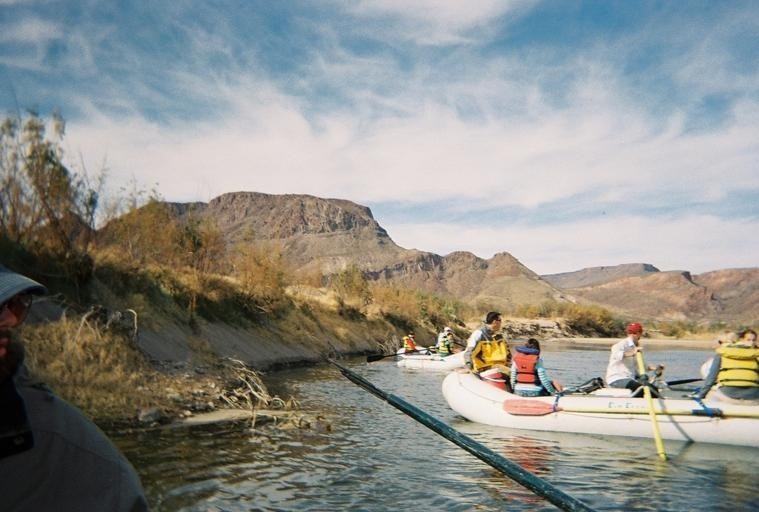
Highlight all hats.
[409,332,416,337]
[0,267,49,307]
[444,326,451,331]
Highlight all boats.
[441,369,759,449]
[396,345,466,370]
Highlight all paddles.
[367,346,439,362]
[504,400,758,416]
[328,358,595,512]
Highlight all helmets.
[625,323,641,333]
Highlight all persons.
[690,330,759,401]
[605,321,665,398]
[402,331,420,355]
[437,326,456,357]
[462,311,562,396]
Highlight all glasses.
[496,320,501,322]
[8,294,32,316]
